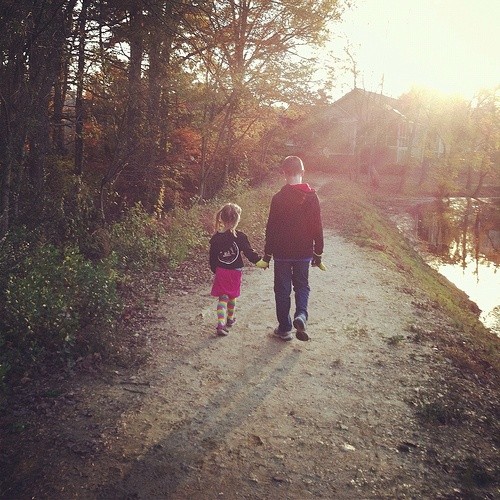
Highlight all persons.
[262,156,323,341]
[210,204,268,337]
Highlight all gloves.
[256,259,269,268]
[311,250,322,268]
[262,253,273,270]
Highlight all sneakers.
[273,328,293,340]
[293,313,310,341]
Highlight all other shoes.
[228,318,236,325]
[217,327,230,336]
